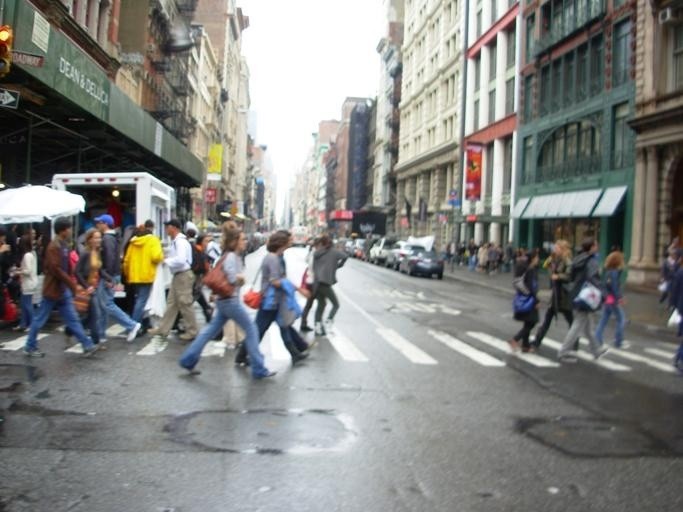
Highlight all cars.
[291,233,306,247]
[197,231,266,266]
[332,234,444,279]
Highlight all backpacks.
[190,244,207,275]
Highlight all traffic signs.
[0,89,18,109]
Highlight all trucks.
[50,171,177,300]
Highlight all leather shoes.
[147,328,168,337]
[179,330,196,340]
[252,369,278,381]
[179,364,201,376]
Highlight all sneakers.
[23,347,45,358]
[81,342,104,358]
[126,322,142,342]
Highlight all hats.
[163,219,180,228]
[94,213,115,227]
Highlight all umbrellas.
[0,184,87,251]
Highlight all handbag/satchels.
[512,275,530,296]
[604,293,616,305]
[4,298,19,321]
[301,273,312,290]
[573,281,603,311]
[562,282,575,294]
[202,267,238,298]
[243,288,265,309]
[305,269,314,284]
[514,293,535,313]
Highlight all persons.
[1,217,348,382]
[439,236,683,379]
